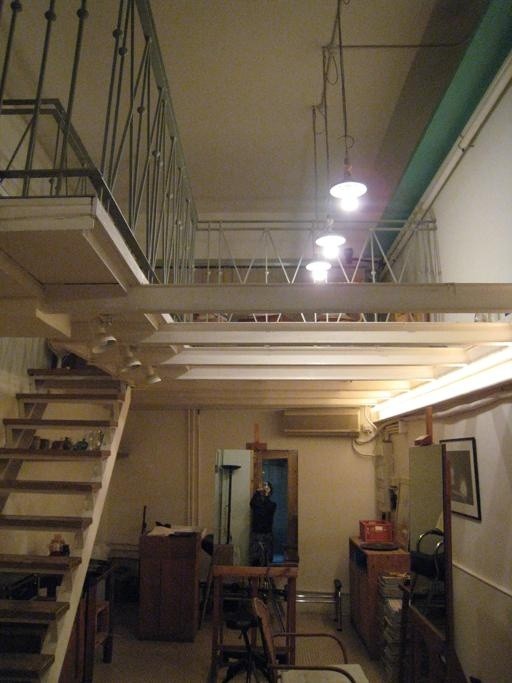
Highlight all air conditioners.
[280,407,364,440]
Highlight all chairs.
[235,595,372,683]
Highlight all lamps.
[315,102,347,261]
[120,345,141,373]
[145,363,163,387]
[307,249,333,286]
[324,0,369,214]
[91,313,117,353]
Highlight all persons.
[249,480,276,567]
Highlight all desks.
[33,555,115,682]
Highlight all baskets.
[360,519,392,541]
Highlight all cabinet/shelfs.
[212,563,296,662]
[348,534,411,656]
[138,525,201,642]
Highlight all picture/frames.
[438,436,481,521]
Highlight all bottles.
[50,533,72,558]
[33,428,104,449]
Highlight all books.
[376,574,411,683]
